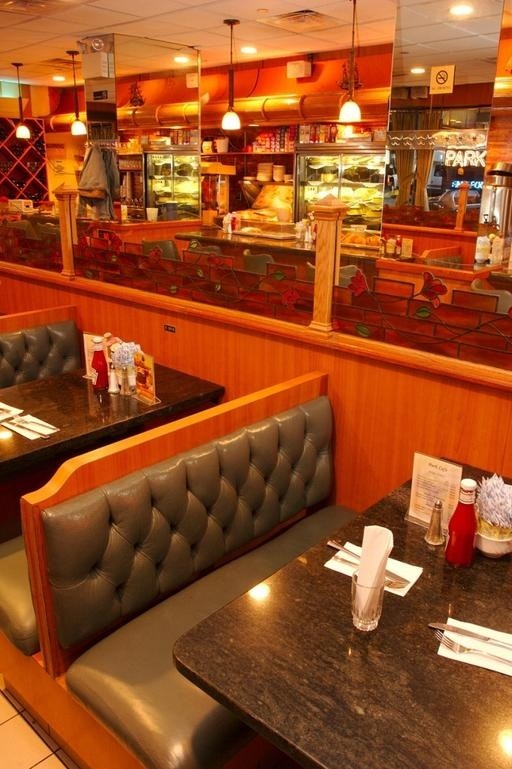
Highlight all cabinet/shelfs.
[201,142,385,229]
[114,144,201,221]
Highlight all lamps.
[338,0,364,125]
[10,48,89,140]
[222,19,243,132]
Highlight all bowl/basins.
[284,175,293,183]
[476,531,512,558]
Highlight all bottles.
[424,500,446,545]
[91,336,132,396]
[224,211,238,234]
[300,216,316,246]
[443,478,478,568]
[385,234,402,258]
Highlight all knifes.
[4,419,50,438]
[428,622,512,651]
[326,539,410,584]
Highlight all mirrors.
[381,2,505,233]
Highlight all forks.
[331,555,408,588]
[433,630,512,667]
[12,414,57,430]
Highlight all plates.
[243,162,286,182]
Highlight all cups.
[350,568,386,632]
